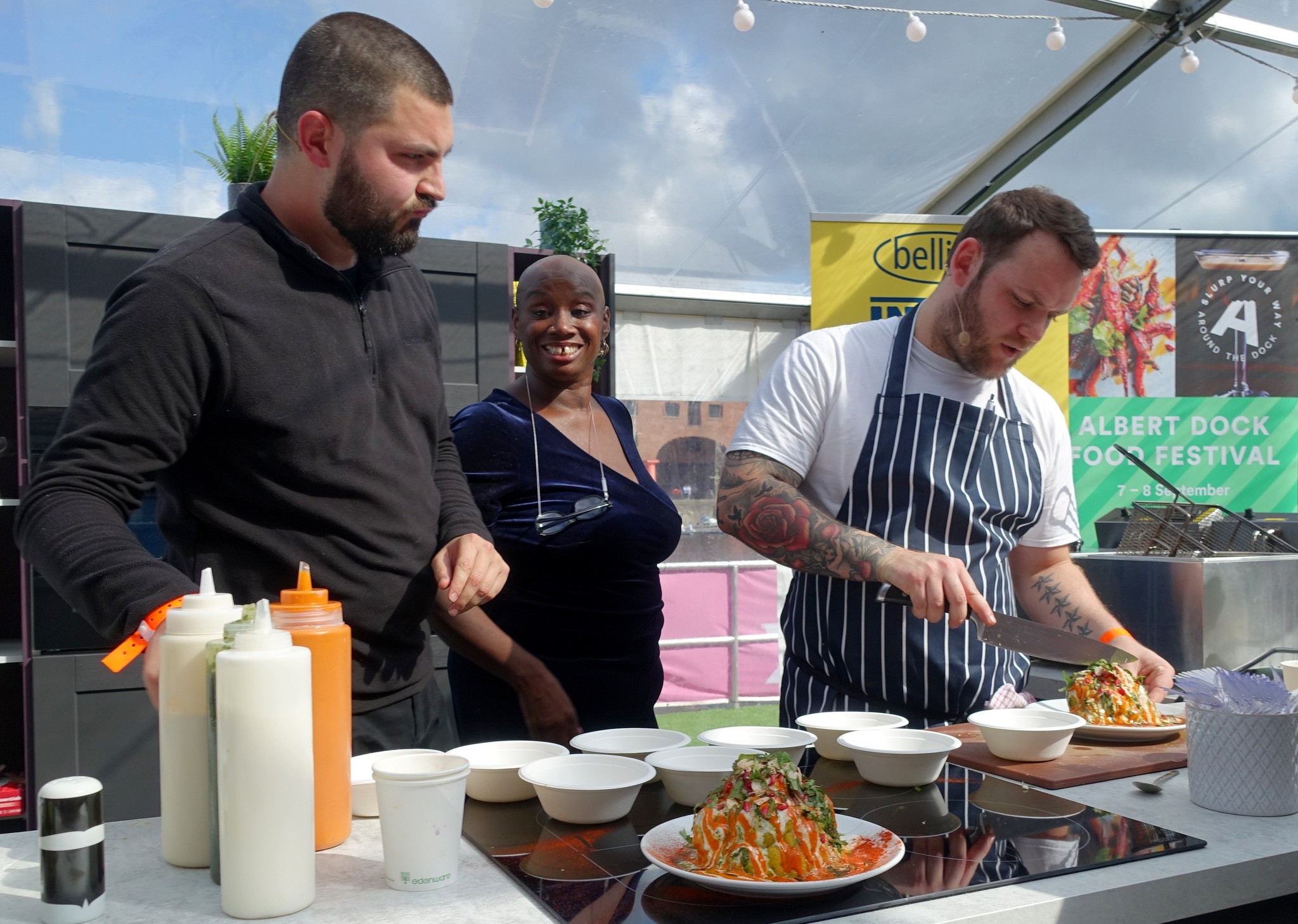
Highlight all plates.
[640,812,907,897]
[1026,698,1186,742]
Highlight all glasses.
[535,495,613,536]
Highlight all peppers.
[1065,232,1176,399]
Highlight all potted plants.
[523,195,610,270]
[195,103,278,210]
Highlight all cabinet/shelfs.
[0,199,26,831]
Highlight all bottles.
[159,566,243,868]
[267,561,351,851]
[217,597,315,922]
[204,603,255,885]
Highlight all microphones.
[953,292,971,345]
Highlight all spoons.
[1131,770,1178,794]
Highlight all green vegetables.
[1058,659,1146,721]
[678,751,848,878]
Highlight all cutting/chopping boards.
[919,717,1188,790]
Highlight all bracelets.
[1099,628,1134,644]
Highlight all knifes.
[876,581,1140,666]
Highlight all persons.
[12,11,507,758]
[426,255,683,755]
[717,187,1176,778]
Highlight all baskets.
[1185,703,1298,817]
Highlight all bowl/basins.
[459,796,654,882]
[350,749,443,818]
[969,775,1085,819]
[967,709,1086,763]
[794,712,910,761]
[447,741,569,803]
[836,728,962,787]
[569,728,693,785]
[809,755,962,838]
[517,754,657,825]
[697,727,817,766]
[646,744,770,806]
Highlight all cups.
[1279,660,1298,692]
[39,775,106,924]
[1184,699,1298,818]
[371,753,470,888]
